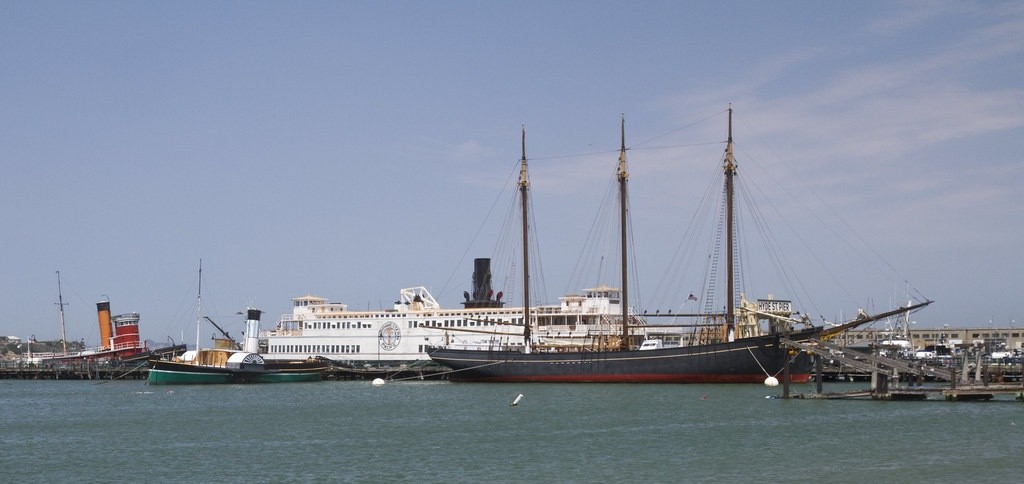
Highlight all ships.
[259,258,652,379]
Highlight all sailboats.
[417,102,935,385]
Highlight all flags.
[688,294,698,301]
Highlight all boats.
[14,268,188,367]
[144,255,330,382]
